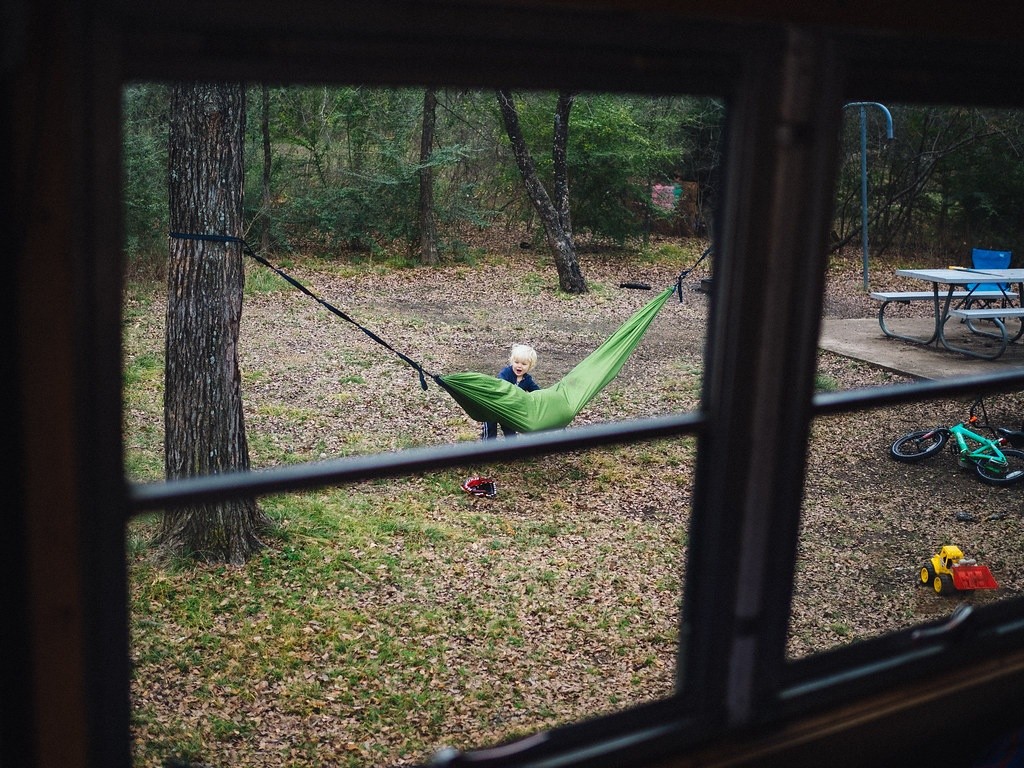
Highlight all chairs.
[961,248,1012,327]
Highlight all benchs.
[870,291,1018,301]
[949,308,1024,319]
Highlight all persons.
[481,344,541,442]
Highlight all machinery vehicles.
[921,543,998,595]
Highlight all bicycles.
[890,395,1024,488]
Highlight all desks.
[879,269,1024,360]
